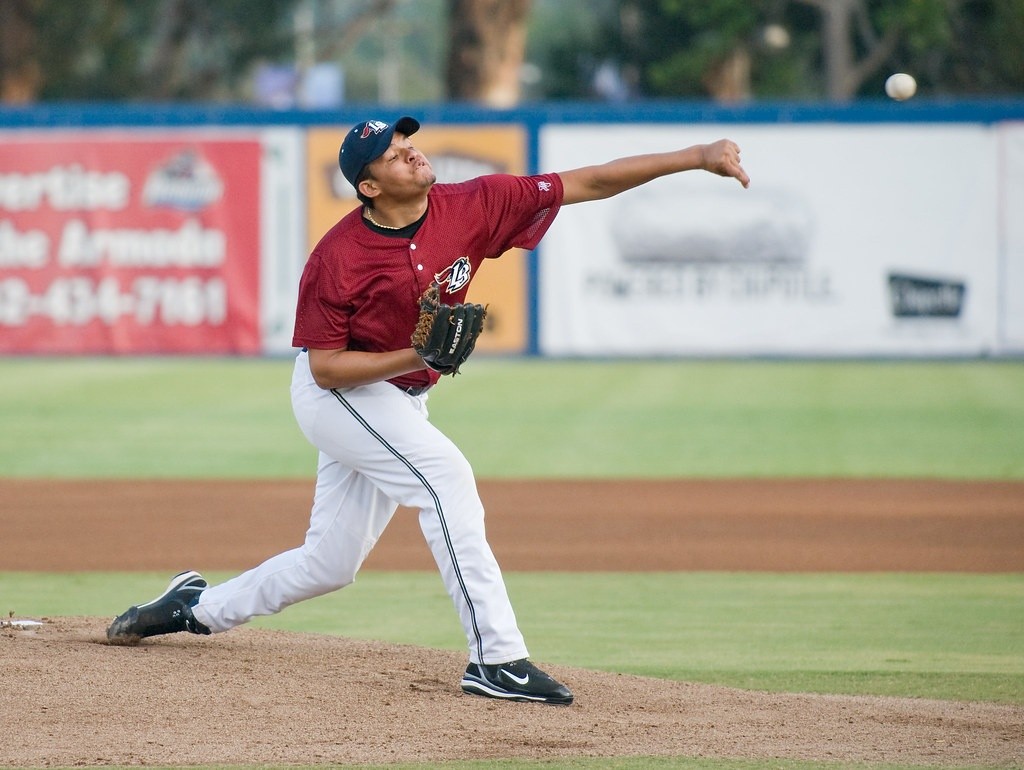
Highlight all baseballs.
[885,72,917,101]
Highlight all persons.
[105,116,750,704]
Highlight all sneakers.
[461,660,573,704]
[105,571,211,644]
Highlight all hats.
[338,116,421,186]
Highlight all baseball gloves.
[409,281,488,376]
